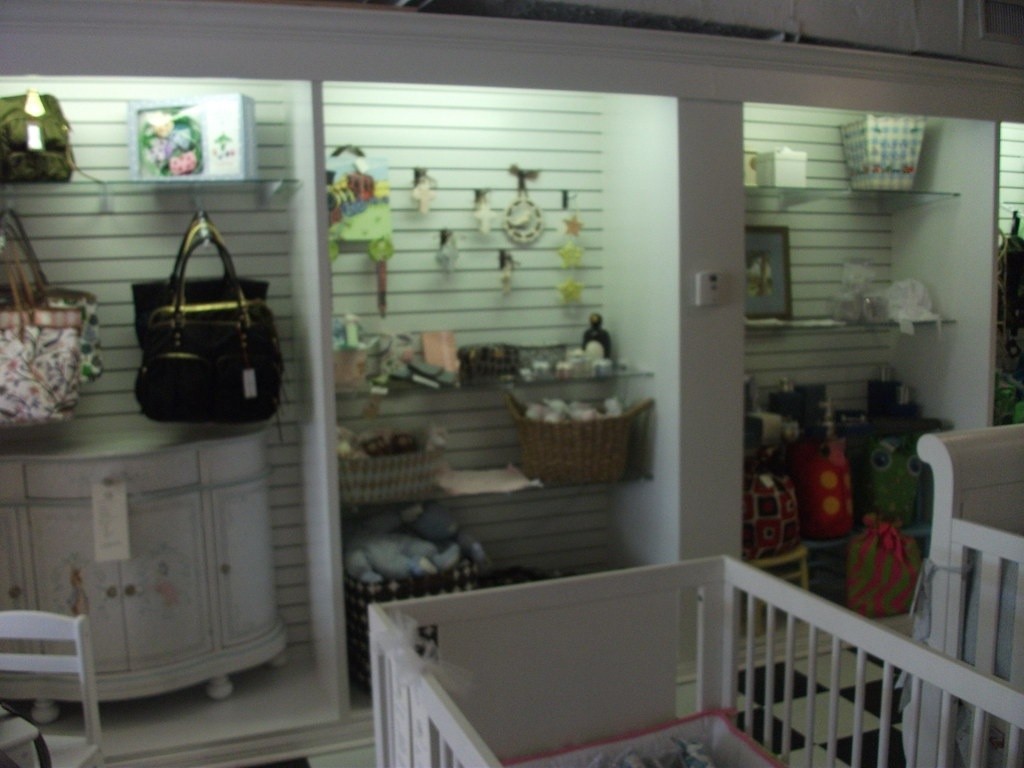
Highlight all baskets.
[333,424,450,504]
[504,390,655,481]
[344,555,493,693]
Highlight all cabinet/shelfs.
[0,427,289,723]
[745,186,960,604]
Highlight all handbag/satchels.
[787,436,855,538]
[738,451,802,561]
[856,432,925,527]
[134,223,283,422]
[0,228,83,424]
[837,508,923,617]
[0,206,104,386]
[130,210,271,348]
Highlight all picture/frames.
[746,226,792,319]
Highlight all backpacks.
[0,87,81,182]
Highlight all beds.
[913,423,1023,768]
[364,555,1024,768]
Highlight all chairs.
[0,610,105,768]
